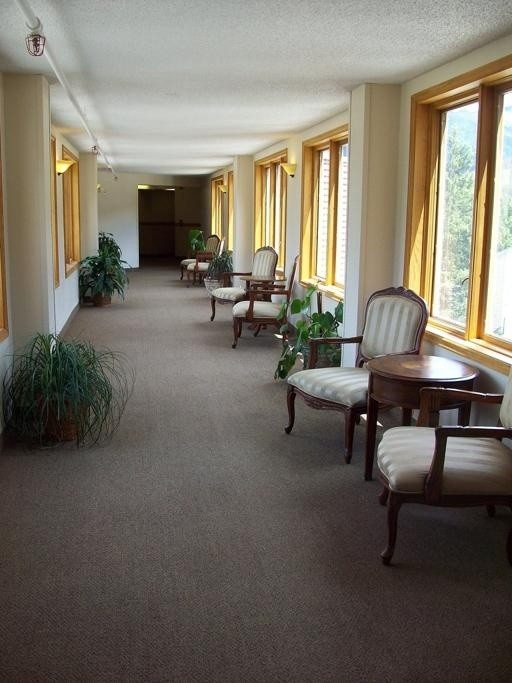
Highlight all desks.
[239,276,287,338]
[364,354,481,483]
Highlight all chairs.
[282,287,429,464]
[376,363,512,563]
[231,256,300,348]
[179,235,221,285]
[210,246,278,328]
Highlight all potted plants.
[77,231,132,307]
[204,248,233,300]
[275,284,343,379]
[0,330,136,450]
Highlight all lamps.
[56,159,75,175]
[218,184,228,193]
[279,162,297,178]
[24,34,45,56]
